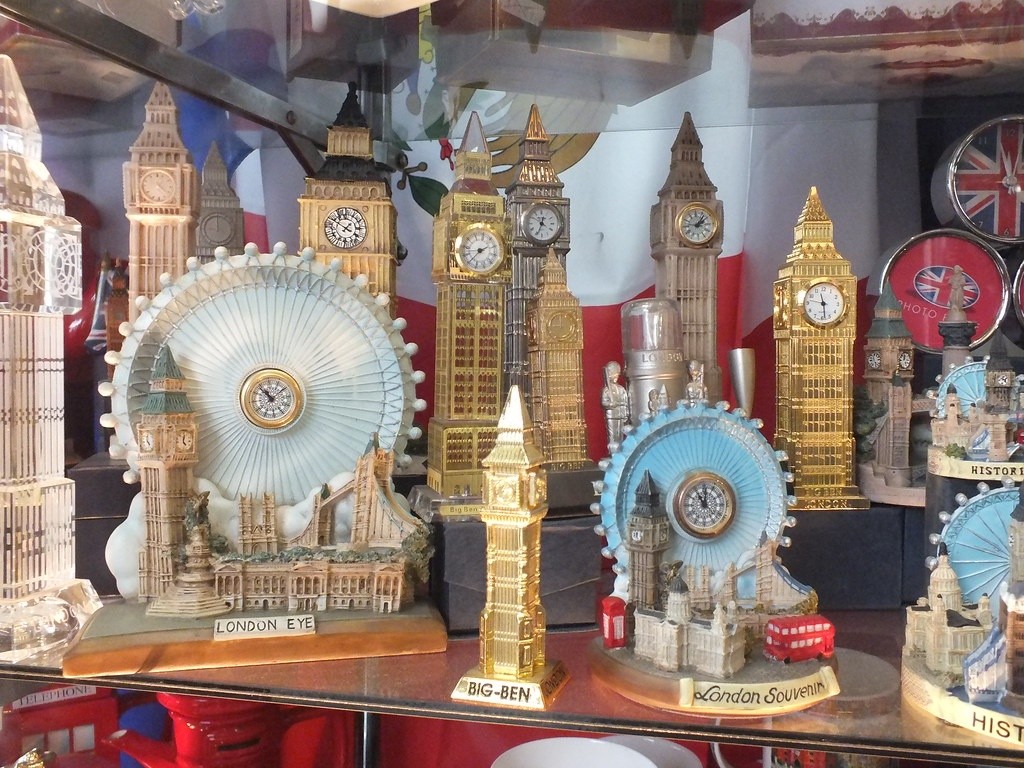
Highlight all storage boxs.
[288,0,1024,109]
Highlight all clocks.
[649,110,724,405]
[592,399,798,592]
[851,278,925,501]
[295,83,398,318]
[99,241,427,491]
[136,346,199,593]
[525,247,596,470]
[1009,479,1024,600]
[774,186,873,509]
[983,318,1014,416]
[502,103,572,425]
[621,471,671,609]
[122,82,199,321]
[410,111,514,516]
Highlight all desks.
[0,467,1024,767]
